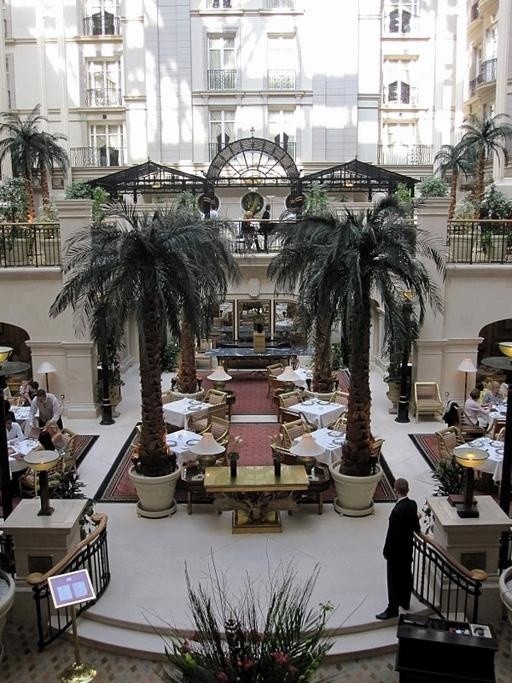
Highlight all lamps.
[453,447,489,517]
[458,358,479,402]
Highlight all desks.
[206,346,298,374]
[203,465,310,534]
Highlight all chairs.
[410,381,506,463]
[131,363,239,517]
[223,215,295,252]
[3,373,76,497]
[268,362,386,516]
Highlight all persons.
[4,413,25,497]
[250,212,264,252]
[29,389,65,450]
[25,428,65,477]
[20,380,40,407]
[210,205,221,220]
[260,204,271,222]
[376,478,422,620]
[241,208,254,249]
[464,360,508,443]
[4,398,18,421]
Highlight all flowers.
[146,545,370,683]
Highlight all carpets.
[408,433,501,495]
[91,369,396,503]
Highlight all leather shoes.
[376,604,410,619]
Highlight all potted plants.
[271,206,346,402]
[135,196,240,405]
[0,177,33,262]
[266,195,458,517]
[477,191,512,262]
[37,198,61,267]
[50,195,241,518]
[449,201,476,261]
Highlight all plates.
[301,401,331,405]
[468,441,504,455]
[328,430,345,446]
[187,400,202,411]
[166,439,200,447]
